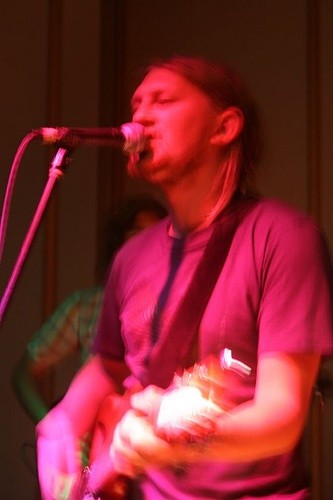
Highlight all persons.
[37,56,333,500]
[13,196,168,424]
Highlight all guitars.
[61,351,250,500]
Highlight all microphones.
[39,122,145,154]
